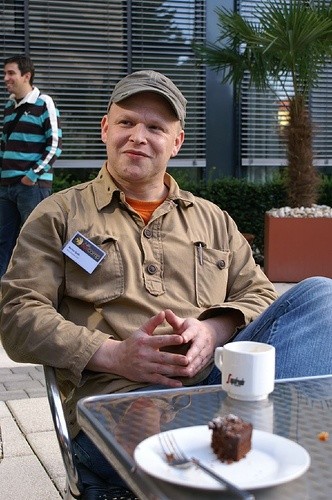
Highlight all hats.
[106,70,188,128]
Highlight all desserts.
[208,414,252,463]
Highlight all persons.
[0,55,62,277]
[0,70,332,500]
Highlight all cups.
[214,341,275,401]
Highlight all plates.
[132,424,311,490]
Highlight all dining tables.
[76,374,331,500]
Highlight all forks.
[155,432,256,500]
